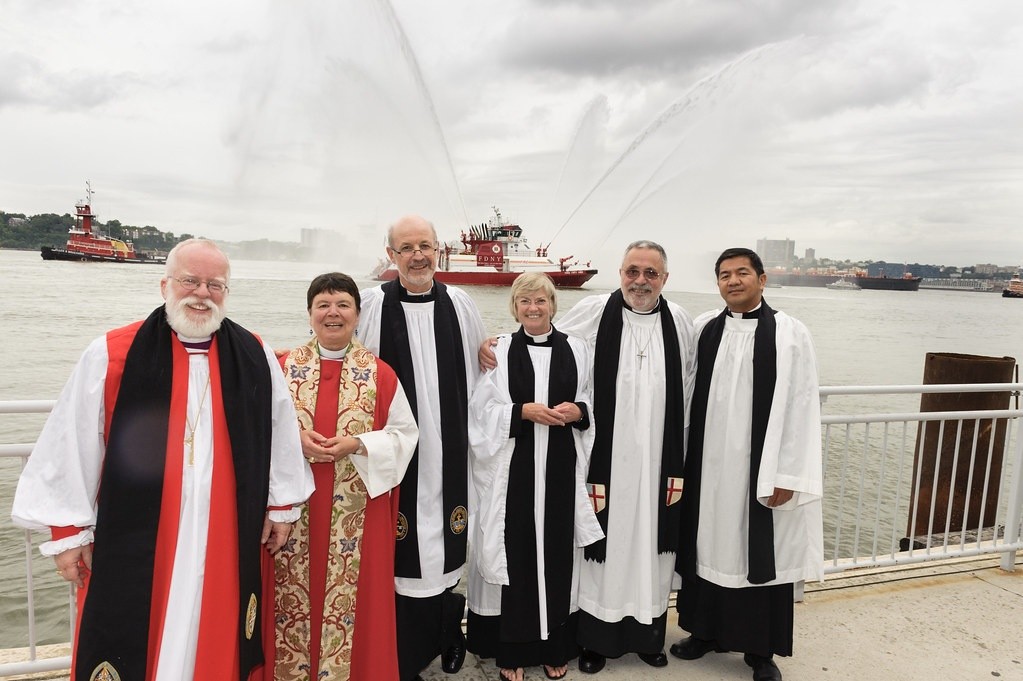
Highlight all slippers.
[500,668,525,681]
[543,664,568,680]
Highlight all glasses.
[178,277,227,294]
[624,269,661,280]
[518,297,549,306]
[394,246,437,258]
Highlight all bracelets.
[576,412,585,423]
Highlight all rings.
[308,457,315,463]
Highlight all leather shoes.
[441,645,466,674]
[578,648,606,674]
[670,637,729,660]
[638,649,668,667]
[744,654,782,681]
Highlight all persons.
[669,248,823,681]
[353,216,488,681]
[469,270,595,681]
[478,240,700,674]
[13,239,315,681]
[247,272,420,681]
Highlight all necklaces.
[183,374,210,465]
[623,305,660,370]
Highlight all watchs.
[353,439,364,455]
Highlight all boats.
[824,277,863,291]
[361,204,600,291]
[36,180,169,266]
[765,282,783,288]
[1003,271,1023,299]
[764,264,927,291]
